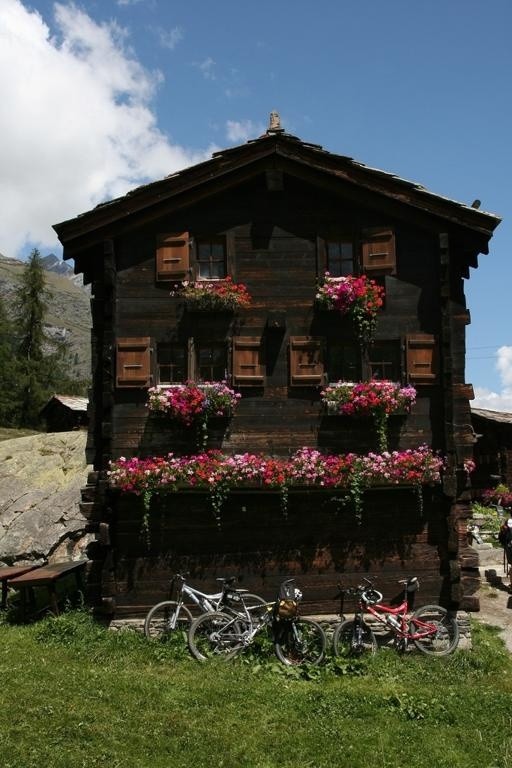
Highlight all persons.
[497,509,511,564]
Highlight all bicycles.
[187,579,328,668]
[332,576,460,659]
[144,570,271,646]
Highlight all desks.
[8,560,89,624]
[0,566,42,614]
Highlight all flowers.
[141,374,242,423]
[168,274,249,319]
[318,377,415,422]
[308,270,384,349]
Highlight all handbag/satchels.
[225,591,241,606]
[275,587,303,622]
